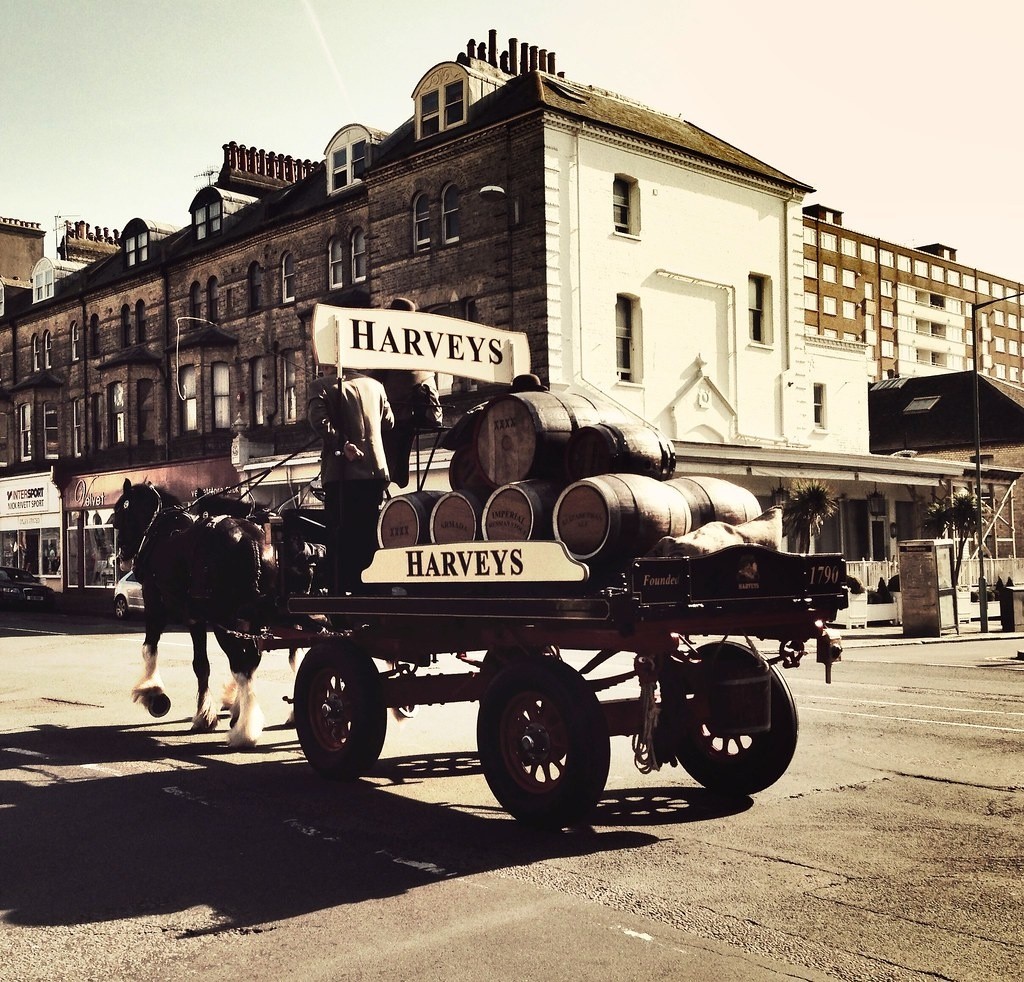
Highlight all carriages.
[104,473,851,827]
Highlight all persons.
[306,298,440,595]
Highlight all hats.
[385,299,415,311]
[508,374,548,392]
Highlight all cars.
[0,566,55,610]
[110,571,148,619]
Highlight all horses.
[111,477,330,749]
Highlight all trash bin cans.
[1000,586,1024,632]
